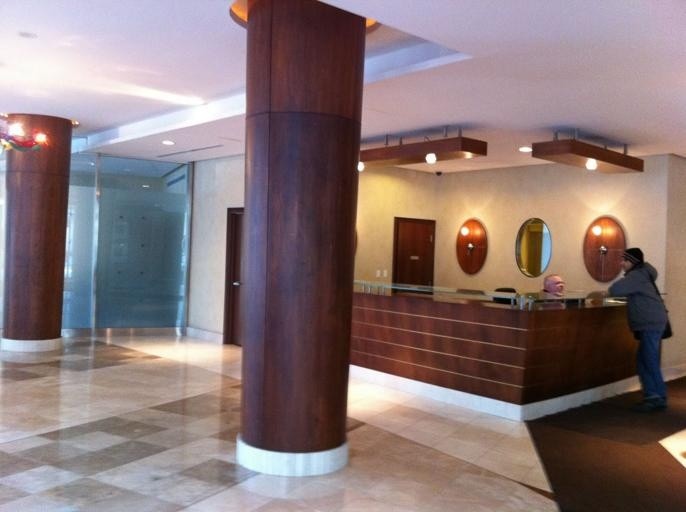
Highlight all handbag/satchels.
[633,318,675,343]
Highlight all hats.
[621,248,644,265]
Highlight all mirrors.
[514,218,552,278]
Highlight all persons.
[608,246,669,414]
[524,275,564,305]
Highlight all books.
[603,296,628,304]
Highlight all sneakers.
[632,395,668,414]
